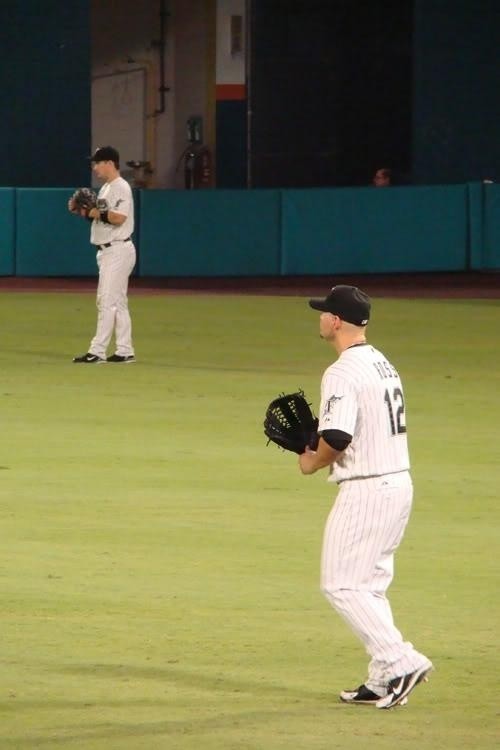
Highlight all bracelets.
[100,209,110,224]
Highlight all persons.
[67,146,138,364]
[374,168,392,186]
[299,284,434,709]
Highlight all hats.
[86,147,119,164]
[308,284,373,329]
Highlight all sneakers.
[71,350,107,366]
[339,661,435,710]
[105,352,137,365]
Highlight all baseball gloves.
[74,188,98,218]
[263,387,319,455]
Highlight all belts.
[95,236,132,251]
